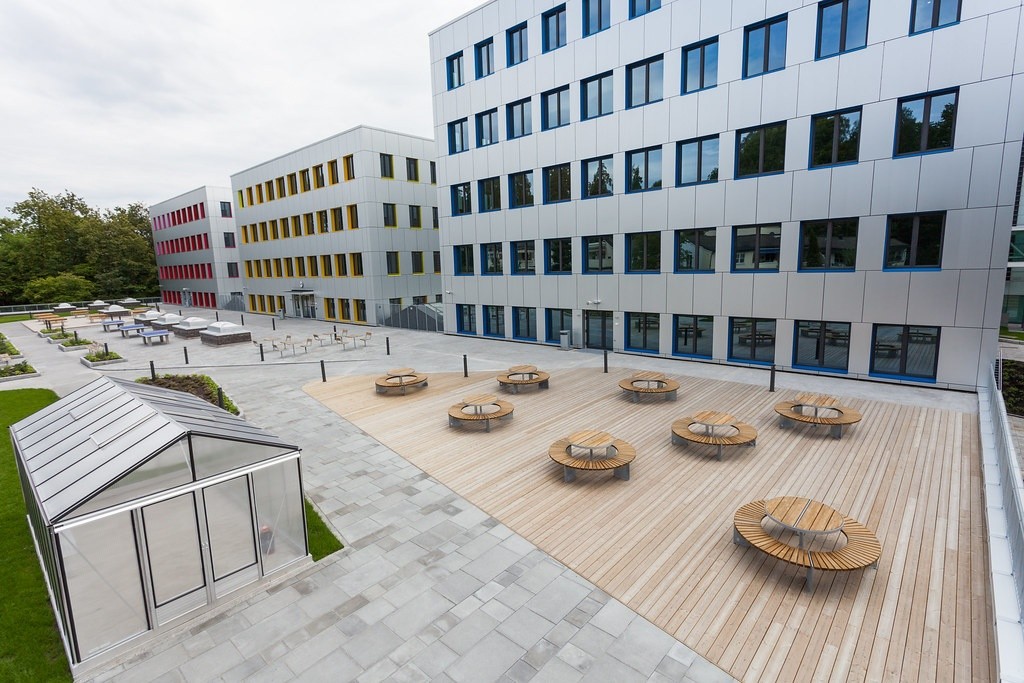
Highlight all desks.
[509,365,537,381]
[346,333,363,349]
[757,328,772,337]
[567,430,615,461]
[910,327,927,334]
[734,319,747,326]
[632,370,665,387]
[831,328,848,337]
[764,496,844,548]
[264,335,281,352]
[463,392,498,415]
[679,321,693,328]
[322,331,336,345]
[691,410,737,438]
[281,339,303,356]
[793,391,839,418]
[31,308,174,346]
[647,316,659,321]
[386,368,416,383]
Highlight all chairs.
[252,329,372,358]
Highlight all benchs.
[448,400,515,433]
[671,417,758,463]
[496,370,550,394]
[547,437,636,484]
[733,499,881,592]
[618,378,681,404]
[635,321,936,358]
[774,400,863,440]
[374,372,428,396]
